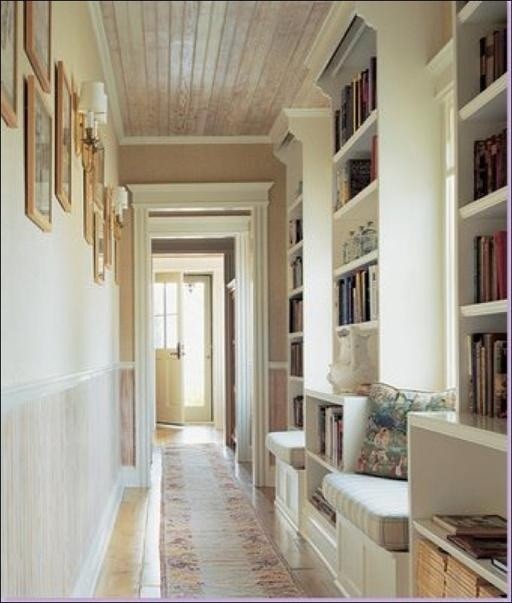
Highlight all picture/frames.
[84,147,119,282]
[0,0,71,232]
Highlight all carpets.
[161,442,307,598]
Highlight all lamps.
[112,187,128,232]
[78,78,108,147]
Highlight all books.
[306,402,344,528]
[328,54,378,326]
[288,214,304,429]
[466,25,508,420]
[410,509,510,601]
